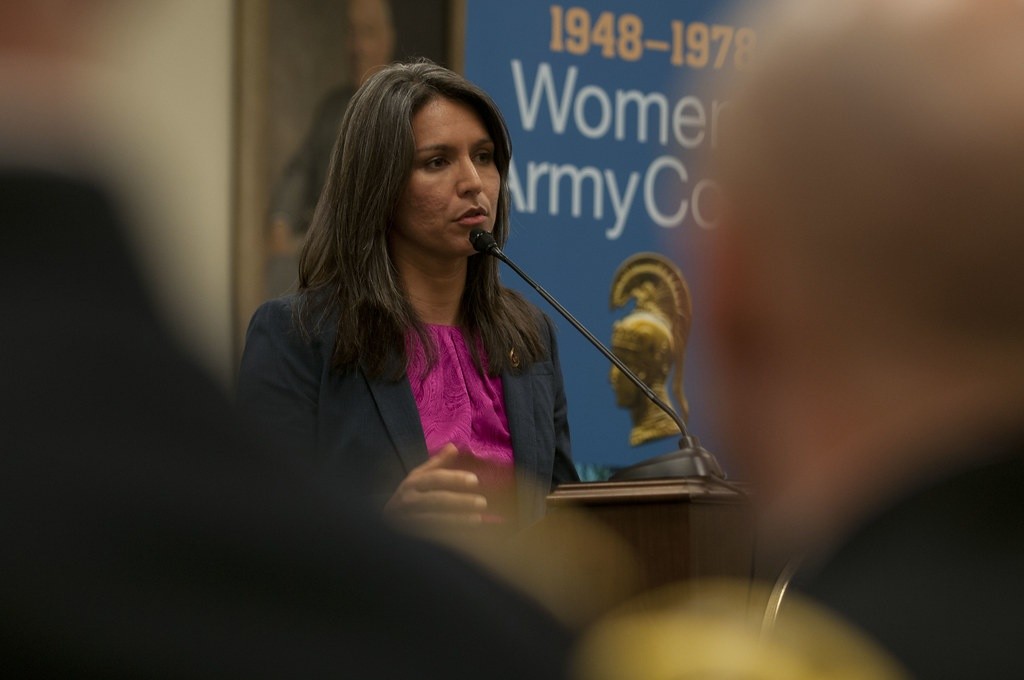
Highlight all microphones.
[467,227,725,481]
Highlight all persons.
[1,1,593,680]
[555,1,1021,679]
[224,62,582,550]
